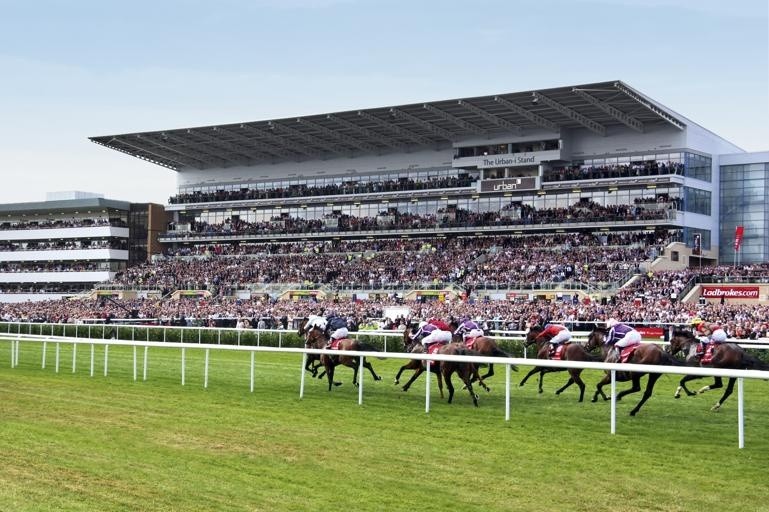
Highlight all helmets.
[691,318,703,325]
[606,318,619,330]
[461,317,469,324]
[419,321,428,329]
[541,321,551,328]
[326,314,334,320]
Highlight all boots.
[423,343,428,353]
[695,341,704,358]
[614,346,621,363]
[548,342,555,356]
[325,337,334,348]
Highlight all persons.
[0,161,769,356]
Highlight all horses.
[584,326,689,417]
[518,325,601,404]
[394,317,517,408]
[666,329,768,411]
[304,323,384,392]
[297,316,339,378]
